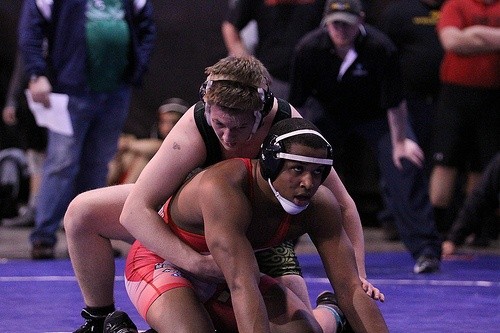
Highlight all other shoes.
[385,226,399,240]
[465,232,489,247]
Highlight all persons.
[18,0,161,260]
[103,118,389,333]
[289,0,441,273]
[64,55,385,333]
[424,0,500,254]
[1,98,188,228]
[222,0,329,123]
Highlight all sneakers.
[413,253,440,274]
[71,306,116,333]
[103,311,139,333]
[316,291,354,333]
[32,245,53,258]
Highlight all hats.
[324,0,362,26]
